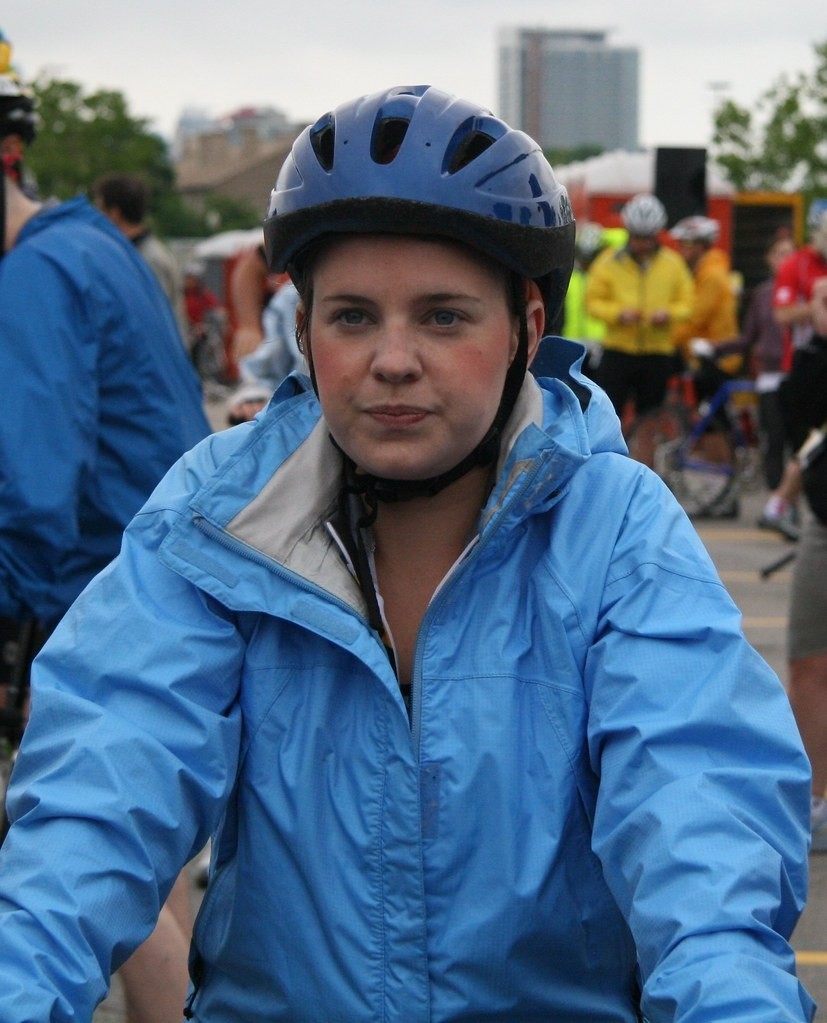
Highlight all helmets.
[622,193,668,235]
[573,223,603,262]
[263,84,575,326]
[668,215,721,241]
[0,28,41,147]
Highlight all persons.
[0,62,213,1022]
[82,170,826,864]
[0,81,816,1022]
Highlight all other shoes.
[756,510,799,540]
[810,800,827,851]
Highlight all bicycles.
[624,337,800,528]
